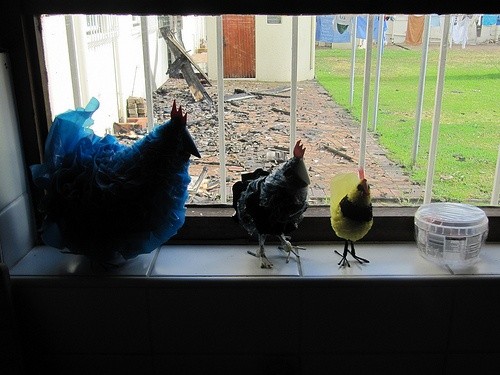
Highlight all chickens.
[329,172,374,267]
[30,100,201,261]
[232,140,310,270]
